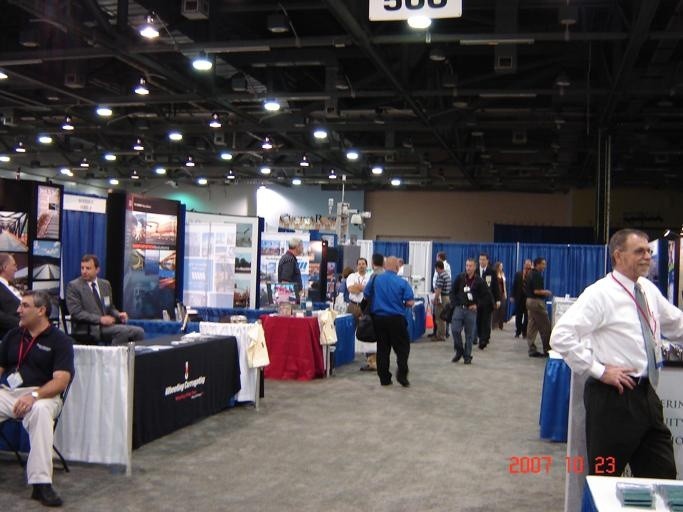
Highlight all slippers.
[360,362,376,371]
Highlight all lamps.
[189,48,215,72]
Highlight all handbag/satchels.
[356,274,378,343]
[440,298,456,323]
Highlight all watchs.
[30,391,40,401]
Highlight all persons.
[277,238,304,306]
[337,252,551,388]
[0,290,74,507]
[66,254,145,346]
[549,229,683,480]
[0,253,23,342]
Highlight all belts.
[628,375,649,386]
[350,300,361,305]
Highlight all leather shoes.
[427,321,553,364]
[396,376,410,386]
[30,487,63,508]
[381,380,393,386]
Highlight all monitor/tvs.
[266,282,300,312]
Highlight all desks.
[118,330,246,451]
[577,465,683,512]
[408,300,425,340]
[256,309,328,381]
[539,342,573,443]
[325,312,357,368]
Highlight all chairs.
[0,363,76,476]
[55,294,104,345]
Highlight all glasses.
[619,248,654,258]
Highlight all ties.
[634,282,659,391]
[91,282,105,316]
[522,270,529,292]
[482,269,485,280]
[9,282,21,292]
